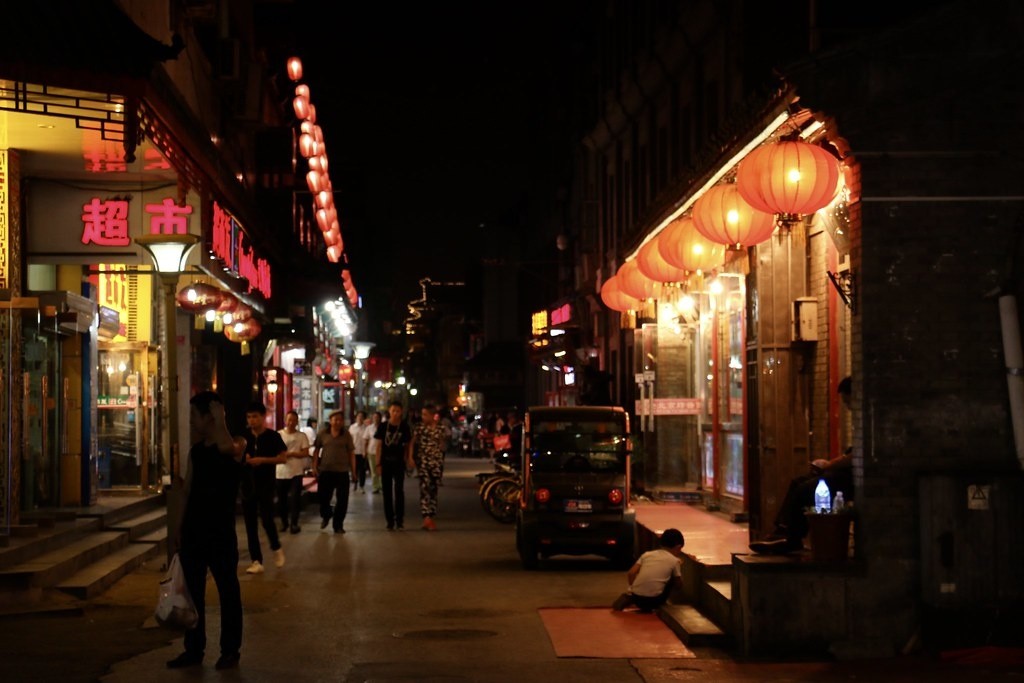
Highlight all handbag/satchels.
[152,552,199,632]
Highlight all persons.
[244,403,524,575]
[613,528,685,615]
[748,376,853,554]
[166,390,243,669]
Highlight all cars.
[517,406,639,572]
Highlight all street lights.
[133,235,201,567]
[349,342,377,415]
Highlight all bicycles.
[475,449,523,522]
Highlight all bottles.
[815,479,831,515]
[832,491,844,514]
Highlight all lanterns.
[339,365,352,385]
[178,279,261,356]
[287,58,357,309]
[601,136,844,329]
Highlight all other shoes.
[273,548,285,567]
[386,521,394,530]
[321,510,333,528]
[749,535,788,554]
[396,523,405,531]
[612,593,628,610]
[167,648,204,669]
[247,562,265,573]
[334,525,345,534]
[422,516,437,530]
[214,651,239,667]
[280,523,289,532]
[290,526,301,533]
[360,487,365,494]
